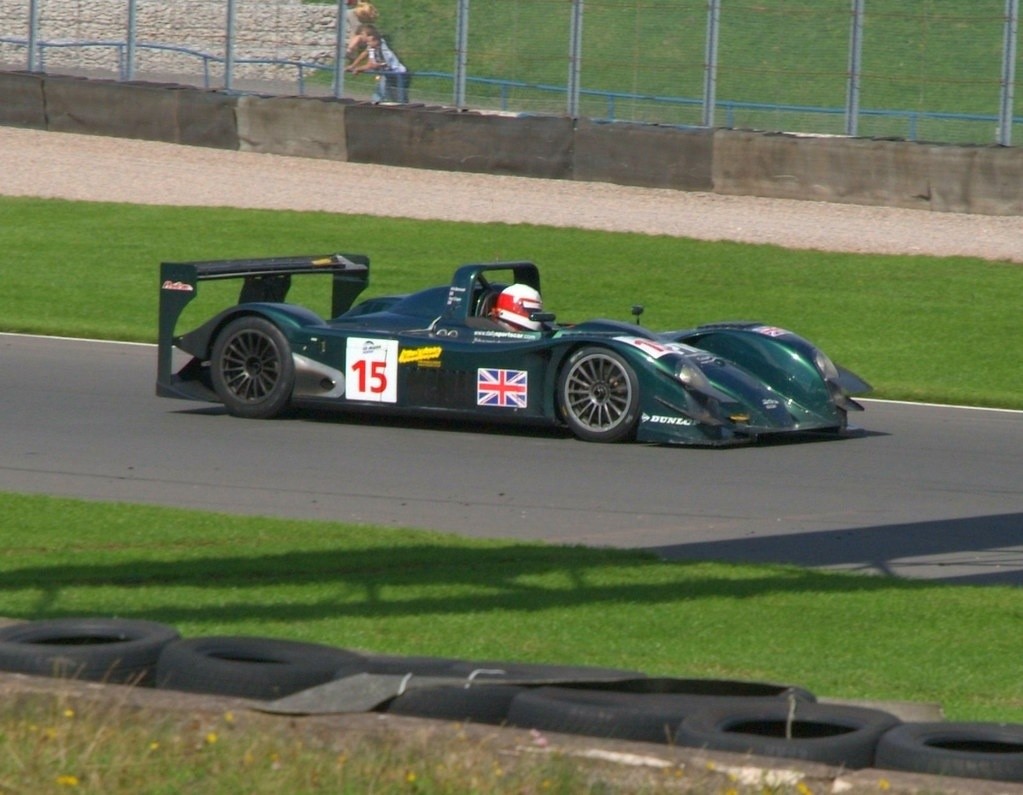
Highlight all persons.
[344,27,389,103]
[366,32,410,104]
[492,283,543,333]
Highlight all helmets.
[496,283,543,331]
